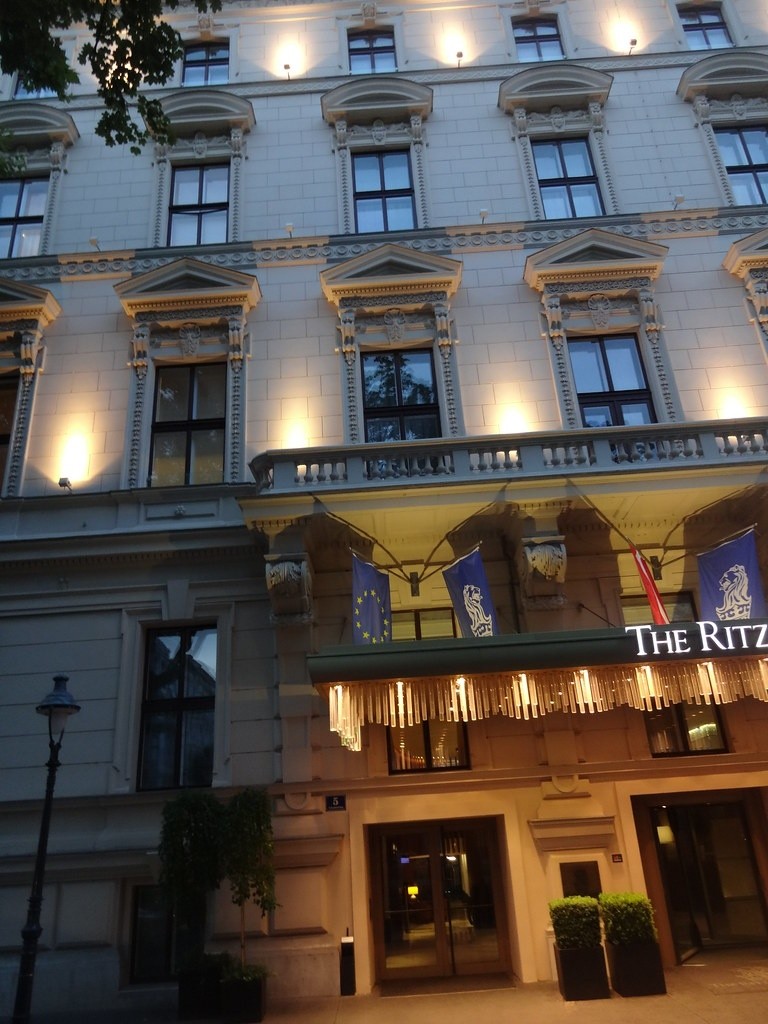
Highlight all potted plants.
[546,895,611,1003]
[600,893,667,999]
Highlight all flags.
[343,545,399,648]
[434,550,498,643]
[692,529,767,625]
[626,539,673,628]
